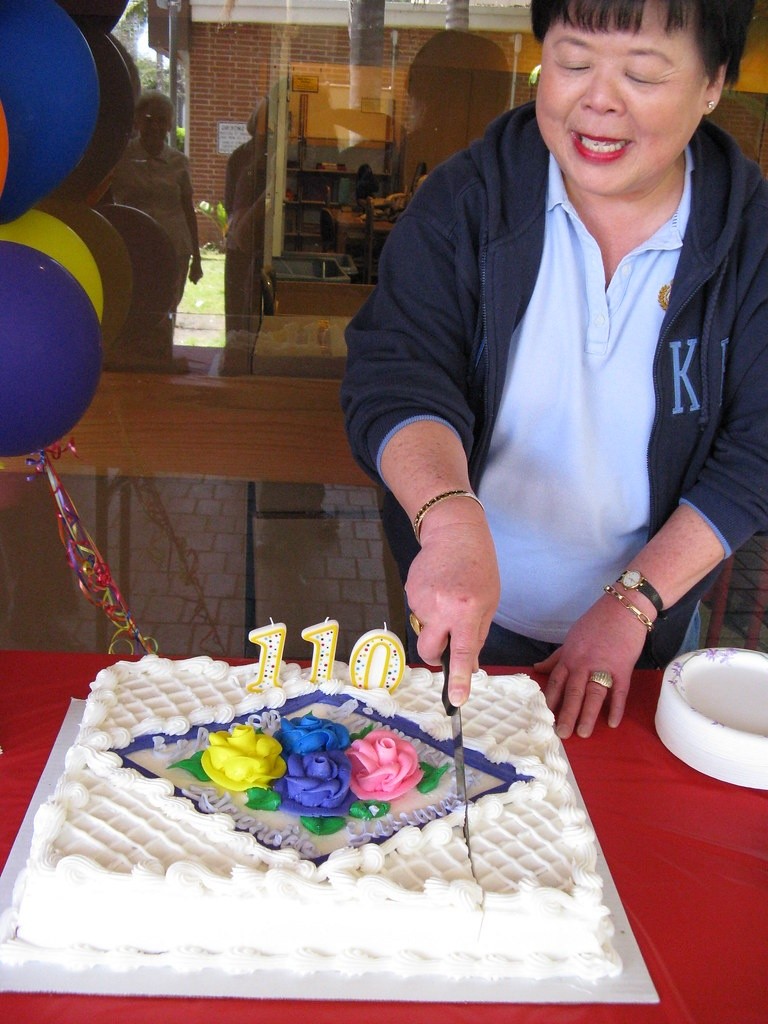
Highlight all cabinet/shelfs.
[284,167,391,252]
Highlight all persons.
[223,30,508,377]
[339,0,768,739]
[112,89,204,372]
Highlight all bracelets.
[414,491,484,541]
[602,582,655,632]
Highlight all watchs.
[617,562,664,611]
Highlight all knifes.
[440,645,470,858]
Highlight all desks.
[0,647,768,1024]
[324,205,377,255]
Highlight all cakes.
[0,653,624,982]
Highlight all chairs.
[363,197,380,285]
[320,208,337,254]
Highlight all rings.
[409,614,424,636]
[589,672,613,689]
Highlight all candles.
[348,622,405,695]
[246,617,288,693]
[301,616,339,684]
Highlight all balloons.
[0,0,106,460]
